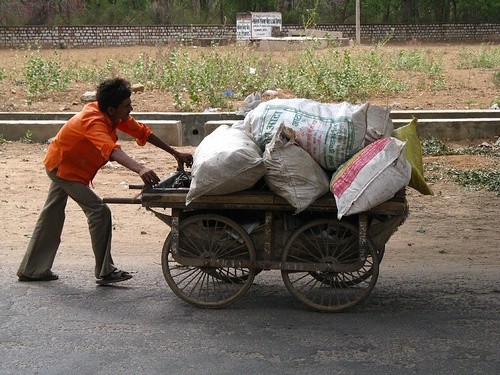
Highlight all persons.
[14,76,195,288]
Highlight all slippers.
[18,270,59,281]
[95,268,132,284]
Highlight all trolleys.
[102,170,410,313]
